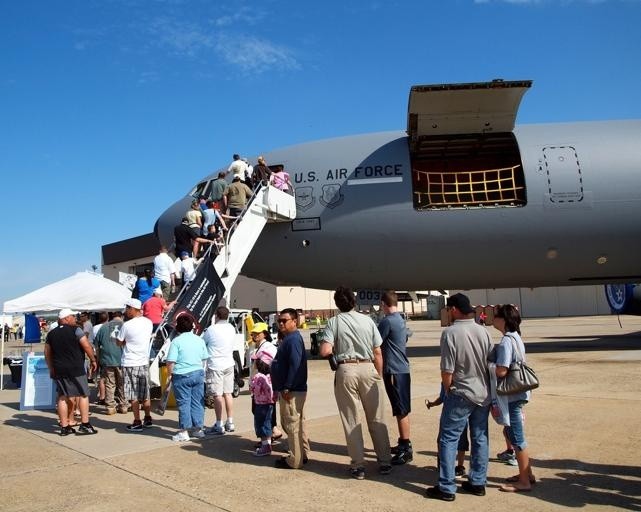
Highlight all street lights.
[91,264,97,273]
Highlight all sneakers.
[154,406,165,416]
[73,376,146,416]
[459,479,490,497]
[379,464,394,474]
[191,429,206,438]
[223,421,236,432]
[126,420,144,432]
[424,485,457,502]
[349,467,366,480]
[390,443,416,465]
[453,464,468,477]
[205,422,225,434]
[60,426,78,436]
[275,457,303,469]
[390,444,401,454]
[171,430,191,441]
[287,454,309,464]
[78,424,99,434]
[496,450,519,467]
[142,416,153,427]
[252,445,273,457]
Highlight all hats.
[249,351,274,367]
[124,298,144,310]
[250,322,270,333]
[58,307,83,319]
[152,287,163,298]
[445,293,478,315]
[180,250,190,257]
[180,153,249,227]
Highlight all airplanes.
[138,74,640,418]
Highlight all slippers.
[505,472,539,482]
[498,481,534,493]
[253,433,284,447]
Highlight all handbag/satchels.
[496,333,546,397]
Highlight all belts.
[337,358,377,365]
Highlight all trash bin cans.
[158,360,177,407]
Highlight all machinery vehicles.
[144,180,277,402]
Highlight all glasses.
[278,317,294,323]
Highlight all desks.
[3,356,23,389]
